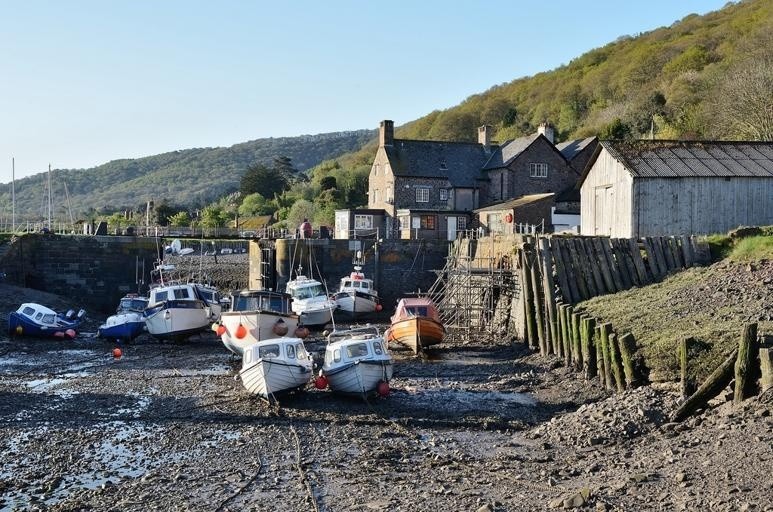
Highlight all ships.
[216,286,303,355]
[144,284,219,343]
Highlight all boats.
[390,297,444,354]
[323,328,395,397]
[8,302,86,340]
[239,335,314,404]
[333,273,381,319]
[96,294,147,345]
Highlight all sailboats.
[195,226,220,320]
[290,225,337,329]
